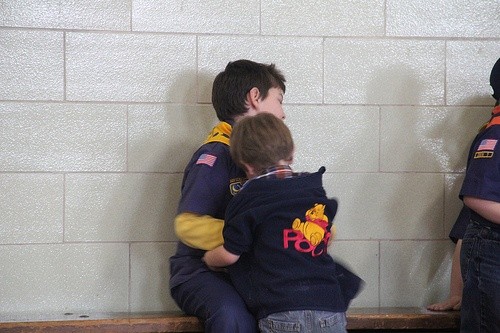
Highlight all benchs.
[1,305,462,333]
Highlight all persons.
[167,57,365,333]
[427,204,472,311]
[457,58,499,332]
[200,107,348,333]
